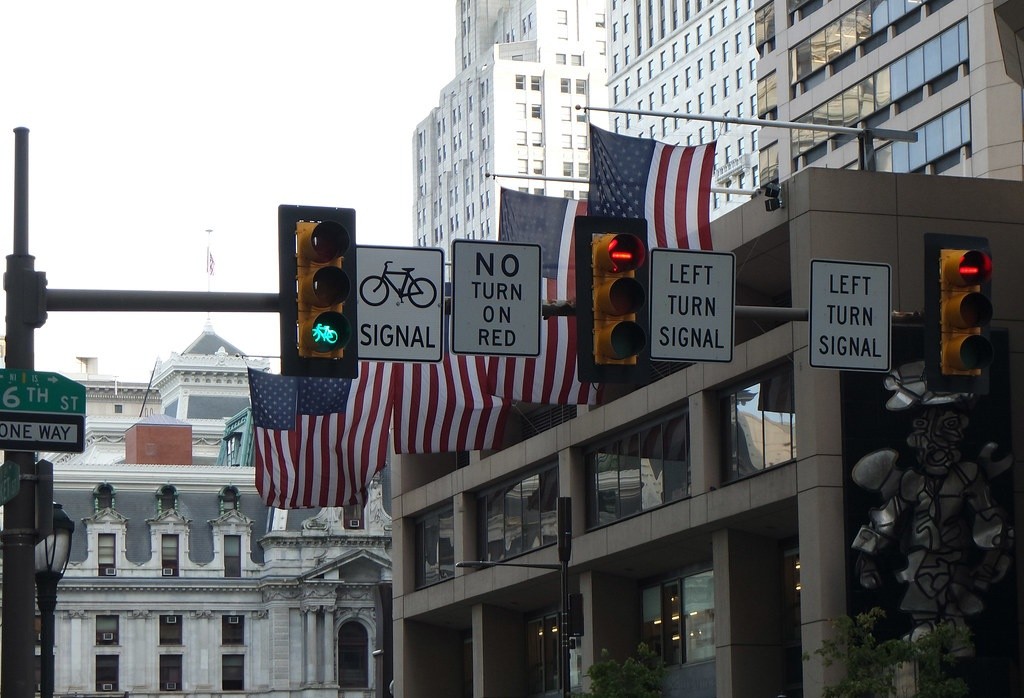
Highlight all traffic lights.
[574,215,651,386]
[279,205,359,379]
[925,232,994,394]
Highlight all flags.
[587,119,717,254]
[390,278,517,455]
[247,365,324,511]
[288,359,393,510]
[484,185,608,407]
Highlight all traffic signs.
[0,369,87,415]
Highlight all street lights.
[455,496,572,698]
[33,501,75,698]
